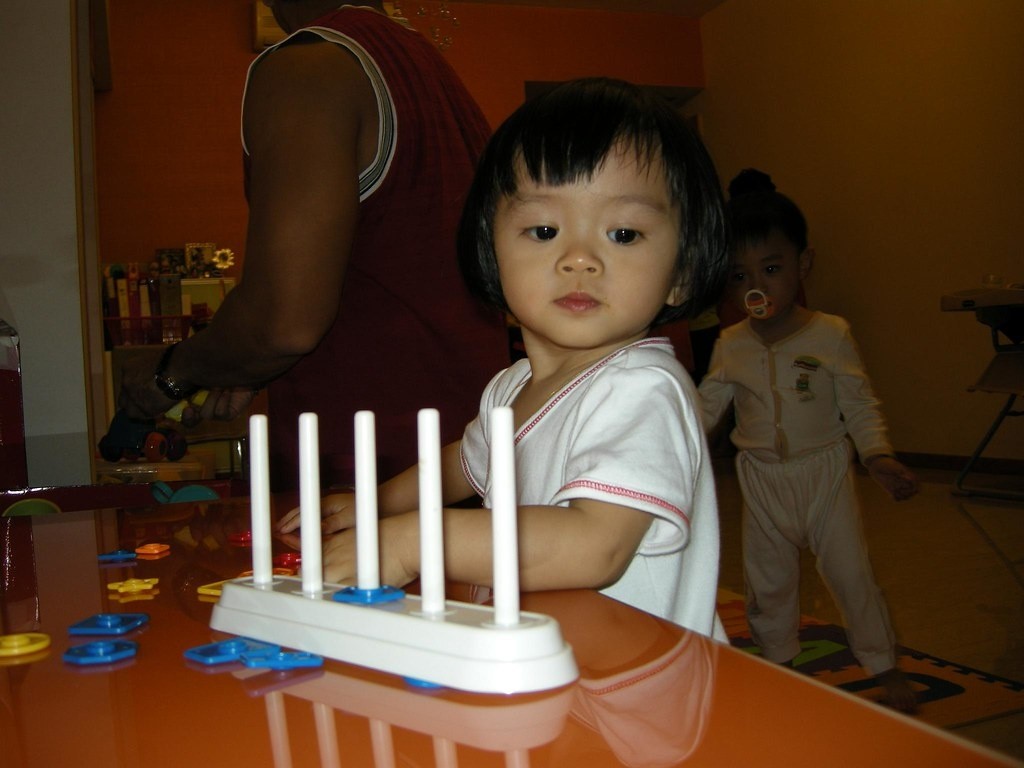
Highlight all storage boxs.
[100,314,193,348]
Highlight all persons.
[698,190,922,712]
[273,78,733,646]
[119,0,511,494]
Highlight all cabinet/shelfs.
[103,345,249,483]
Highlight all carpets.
[713,586,1024,732]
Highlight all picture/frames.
[185,243,217,270]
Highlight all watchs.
[153,343,200,401]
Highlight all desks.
[0,490,1024,768]
